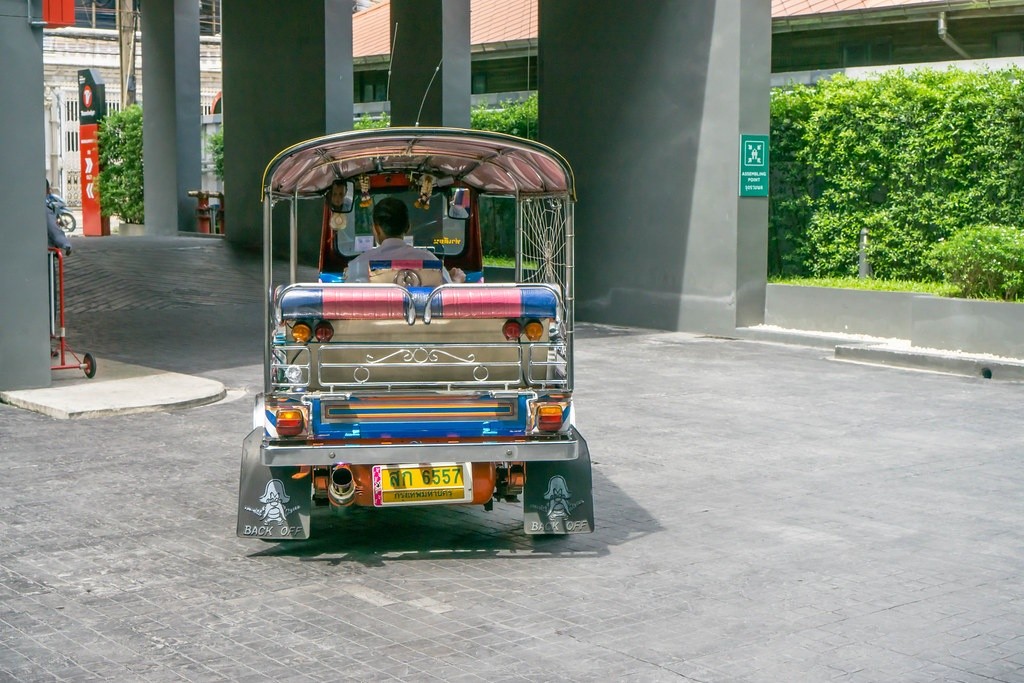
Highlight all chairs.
[369,259,443,286]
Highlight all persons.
[46,180,71,356]
[330,181,351,212]
[345,198,466,286]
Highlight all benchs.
[272,282,562,389]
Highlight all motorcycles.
[49,194,76,233]
